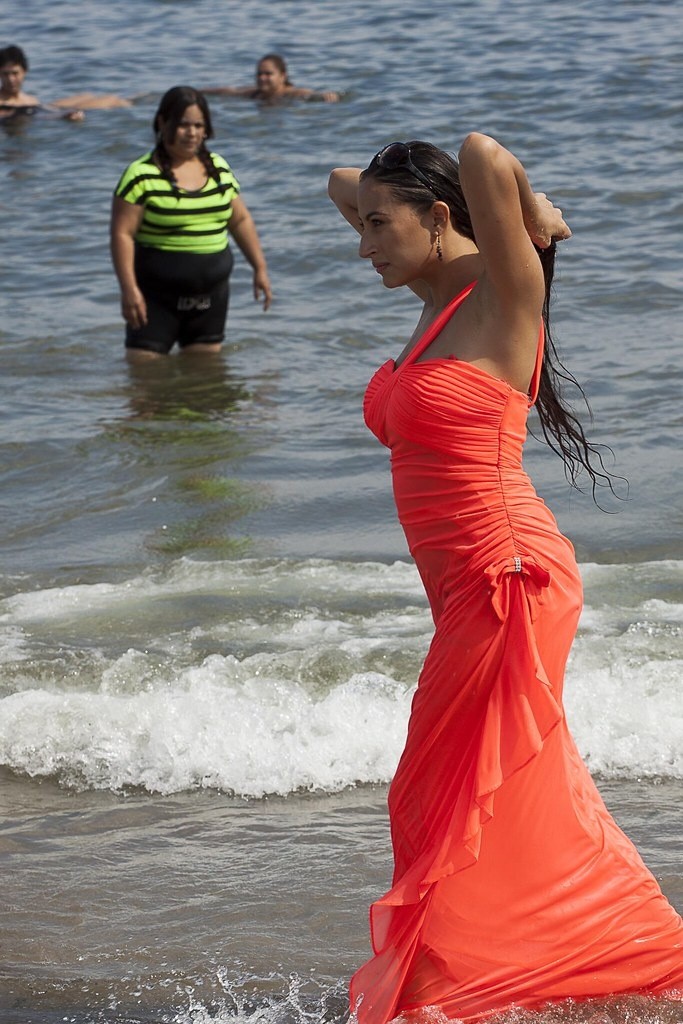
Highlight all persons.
[328,132,683,1023]
[202,54,338,102]
[111,86,272,355]
[0,44,84,120]
